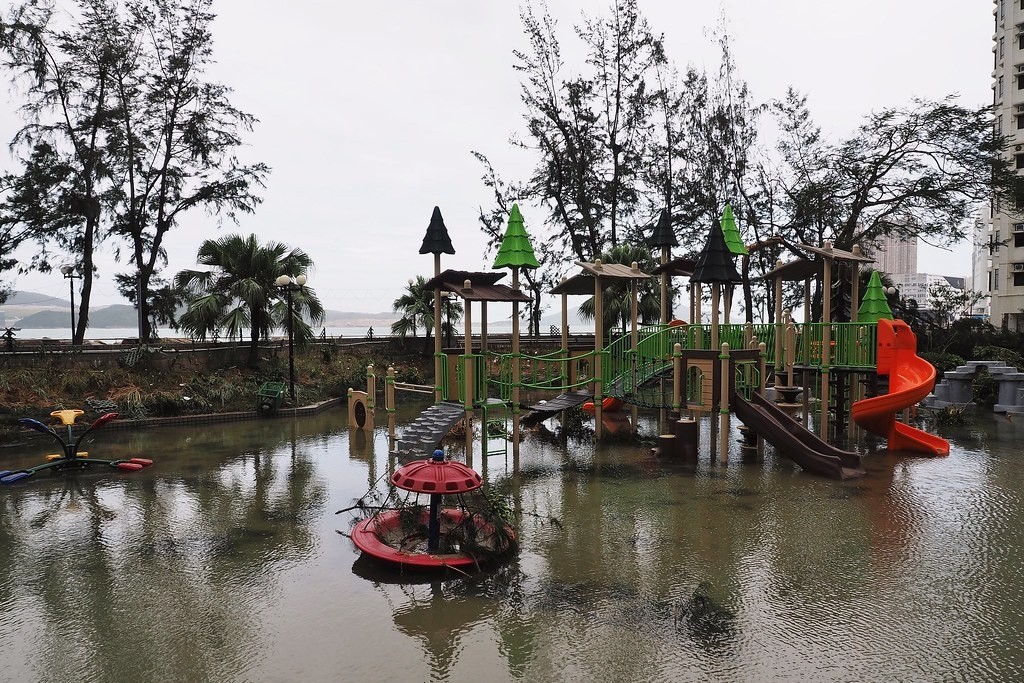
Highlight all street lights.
[276,274,306,400]
[409,278,421,336]
[61,265,84,345]
[442,288,460,347]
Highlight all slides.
[849,354,951,457]
[735,390,868,480]
[582,395,618,412]
